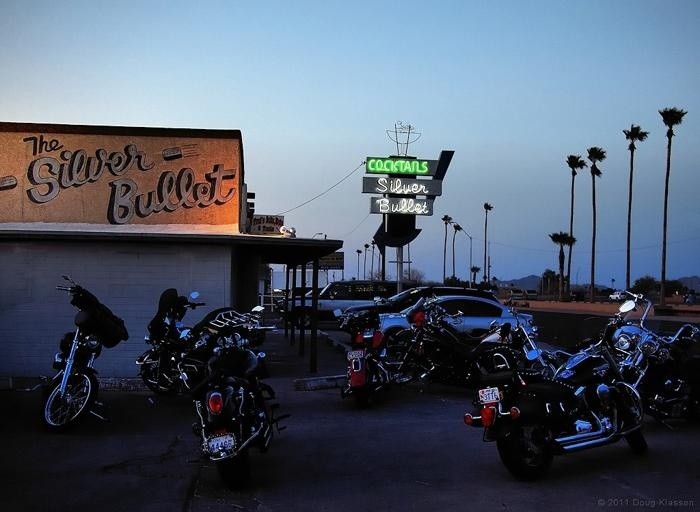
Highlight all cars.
[378,296,539,339]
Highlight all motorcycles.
[388,296,532,386]
[41,277,128,430]
[136,288,289,490]
[331,297,397,405]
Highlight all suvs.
[338,287,501,334]
[276,280,414,328]
